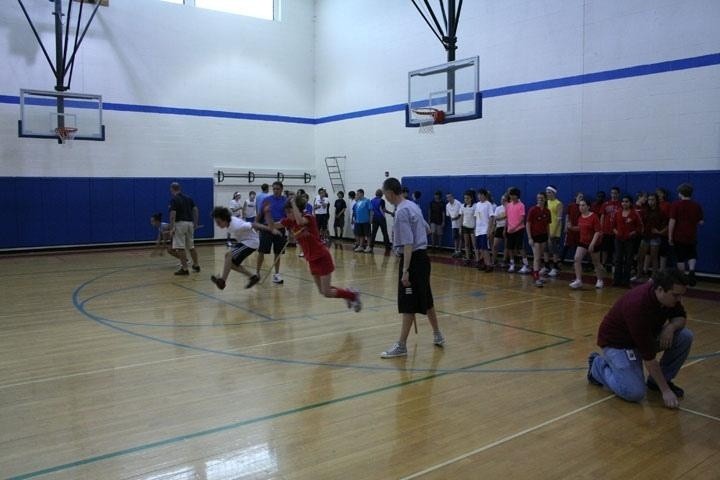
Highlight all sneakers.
[174,268,189,275]
[364,246,373,252]
[355,246,364,251]
[385,248,391,255]
[352,288,361,312]
[298,251,304,257]
[450,250,698,288]
[647,374,684,398]
[587,352,603,387]
[346,287,352,309]
[273,273,284,284]
[381,342,407,358]
[211,275,226,289]
[433,332,444,345]
[245,275,259,290]
[192,264,200,272]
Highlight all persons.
[401,184,704,290]
[150,179,445,358]
[587,269,694,409]
